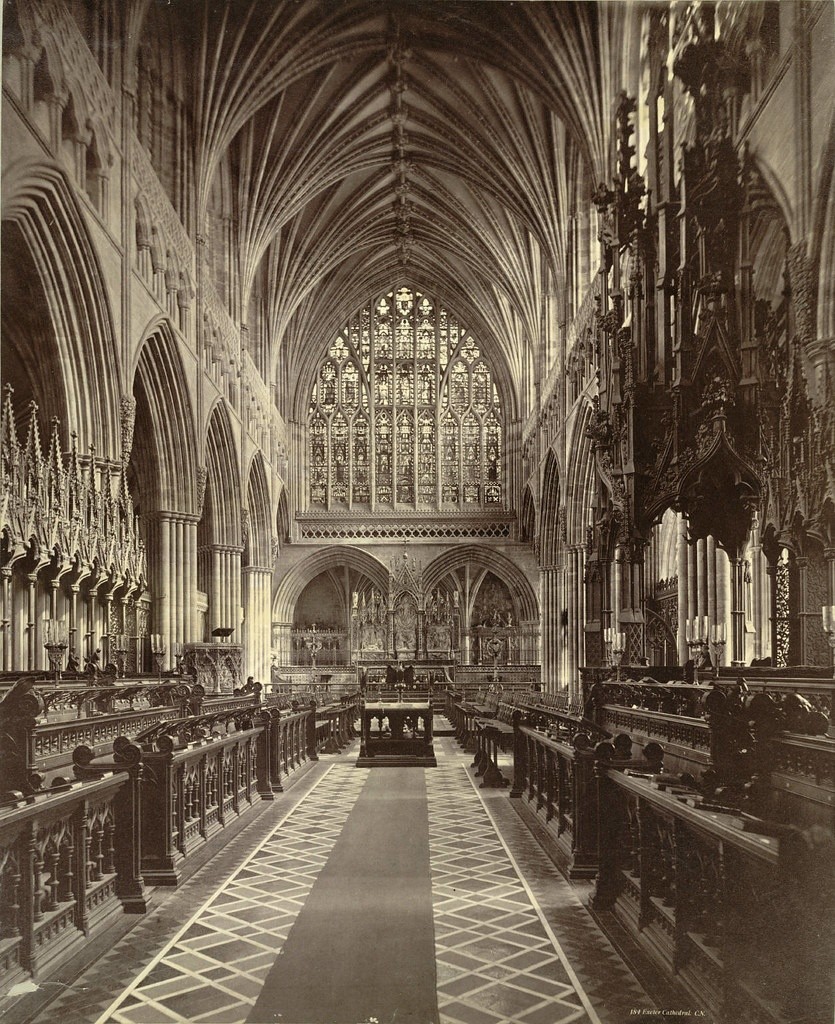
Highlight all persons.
[67,646,79,673]
[240,677,253,695]
[90,649,102,669]
[386,662,415,690]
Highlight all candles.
[717,625,721,640]
[622,633,625,648]
[608,628,611,640]
[712,625,715,638]
[150,634,154,647]
[156,634,159,646]
[695,616,700,636]
[832,606,835,621]
[612,635,616,648]
[822,607,827,621]
[617,633,621,646]
[162,635,165,645]
[612,627,615,635]
[724,623,726,641]
[48,629,63,643]
[686,620,691,636]
[704,615,708,638]
[604,629,607,641]
[116,635,130,650]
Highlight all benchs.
[464,690,568,754]
[262,691,342,753]
